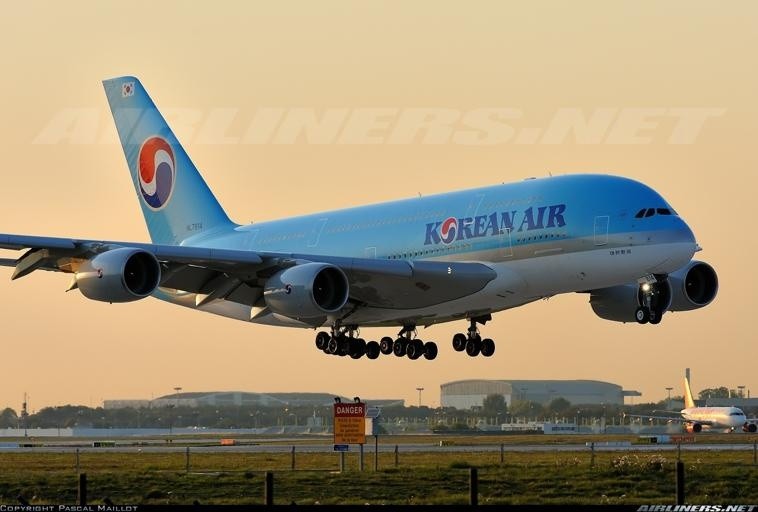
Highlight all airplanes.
[622,369,757,433]
[0,76,719,360]
